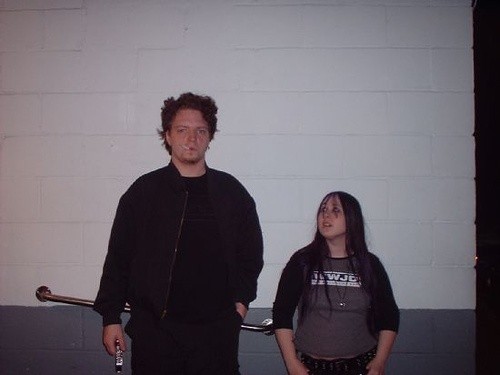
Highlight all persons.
[272,191,400,375]
[92,92,264,375]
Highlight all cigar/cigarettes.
[179,142,190,150]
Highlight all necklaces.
[329,253,350,309]
